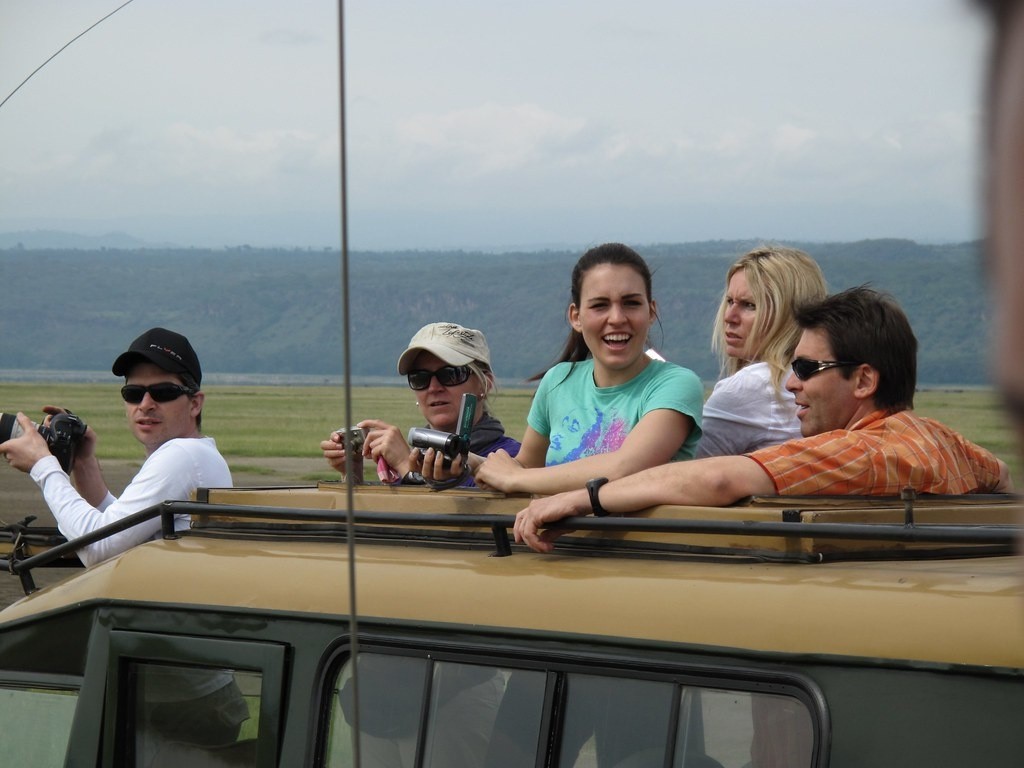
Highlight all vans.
[0,468,1023,768]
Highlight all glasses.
[121,382,194,403]
[791,359,863,380]
[408,365,487,390]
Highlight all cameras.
[0,411,86,475]
[336,426,371,454]
[408,393,478,468]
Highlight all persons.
[513,283,1010,768]
[695,245,830,768]
[0,329,253,768]
[408,245,700,768]
[321,322,524,768]
[967,0,1024,399]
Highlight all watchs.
[586,476,610,517]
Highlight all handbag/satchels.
[343,654,497,737]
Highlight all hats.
[112,329,202,386]
[398,322,493,375]
